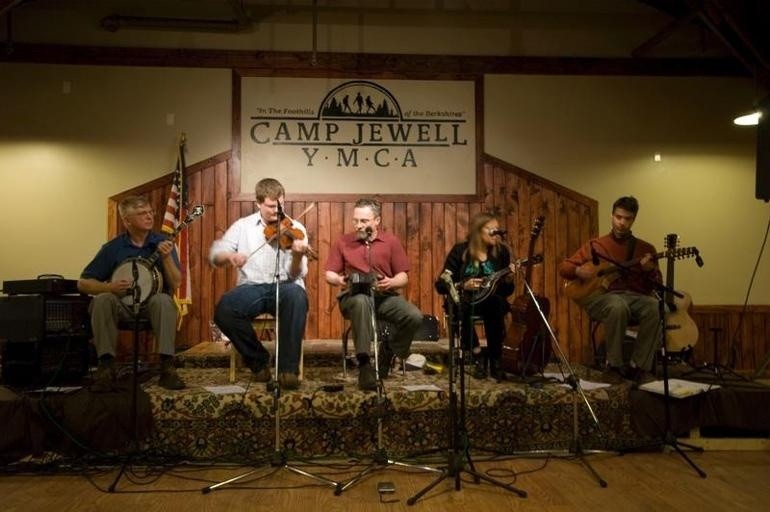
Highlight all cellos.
[499,215,551,377]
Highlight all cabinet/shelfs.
[753,95,770,202]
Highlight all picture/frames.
[231,66,485,202]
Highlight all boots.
[489,349,507,380]
[473,346,489,378]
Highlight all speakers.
[756,114,770,201]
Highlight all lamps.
[733,94,770,126]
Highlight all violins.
[264,218,318,260]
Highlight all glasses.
[129,209,156,217]
[482,224,499,231]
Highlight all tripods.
[619,294,709,479]
[106,289,180,494]
[334,285,445,495]
[407,297,527,505]
[203,218,344,495]
[513,379,624,488]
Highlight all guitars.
[661,234,697,355]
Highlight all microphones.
[131,258,139,280]
[489,229,507,236]
[278,200,285,220]
[365,226,372,236]
[589,247,602,265]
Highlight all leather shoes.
[157,369,184,389]
[92,373,116,390]
[358,367,377,388]
[255,354,271,382]
[625,367,645,382]
[602,366,624,384]
[279,371,301,390]
[378,342,393,377]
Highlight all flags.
[162,139,191,332]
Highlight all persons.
[436,211,515,383]
[209,178,309,389]
[76,194,188,390]
[322,199,423,391]
[560,197,664,380]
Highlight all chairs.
[442,293,489,382]
[116,292,185,332]
[586,311,657,376]
[341,320,406,378]
[220,313,276,383]
